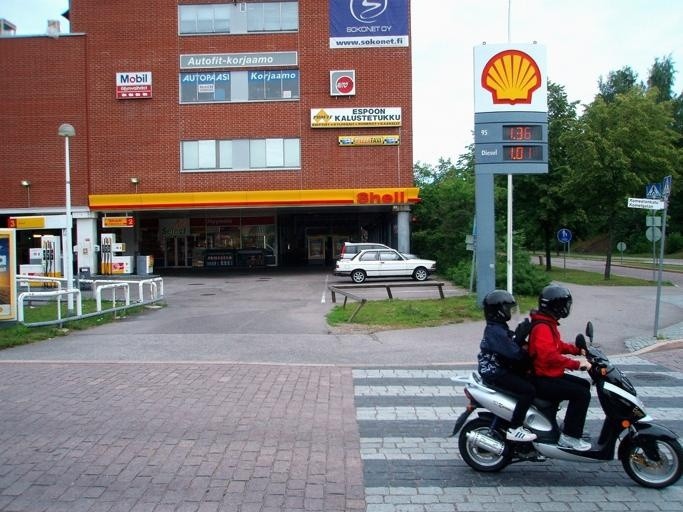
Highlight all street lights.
[57,123,78,318]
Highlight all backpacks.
[512,319,555,372]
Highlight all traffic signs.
[628,196,663,212]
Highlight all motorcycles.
[449,320,682,489]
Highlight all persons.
[477,290,538,442]
[528,285,592,452]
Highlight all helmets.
[538,285,572,318]
[483,290,516,322]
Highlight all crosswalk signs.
[644,182,663,200]
[662,176,672,196]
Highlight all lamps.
[131,177,139,194]
[20,180,32,208]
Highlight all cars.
[335,249,437,281]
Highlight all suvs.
[338,241,420,260]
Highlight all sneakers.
[507,426,537,442]
[557,433,592,451]
